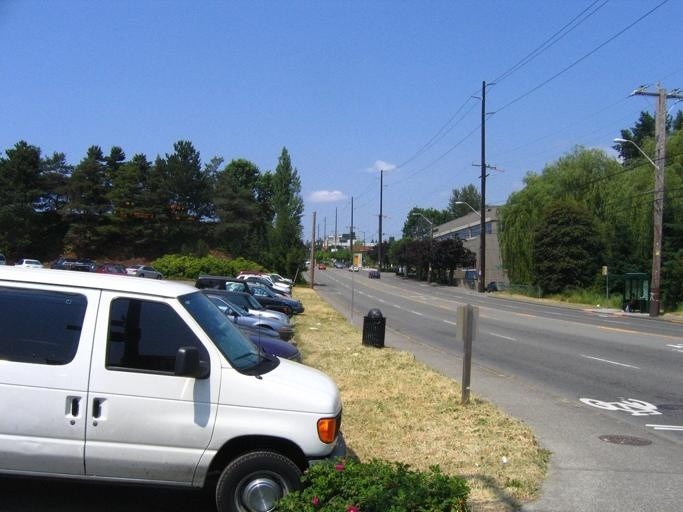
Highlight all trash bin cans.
[363,308,385,347]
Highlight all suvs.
[0,265,343,512]
[463,271,480,288]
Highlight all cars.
[367,270,380,280]
[485,281,503,293]
[0,256,305,371]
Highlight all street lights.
[454,199,484,293]
[611,135,664,320]
[410,211,433,282]
